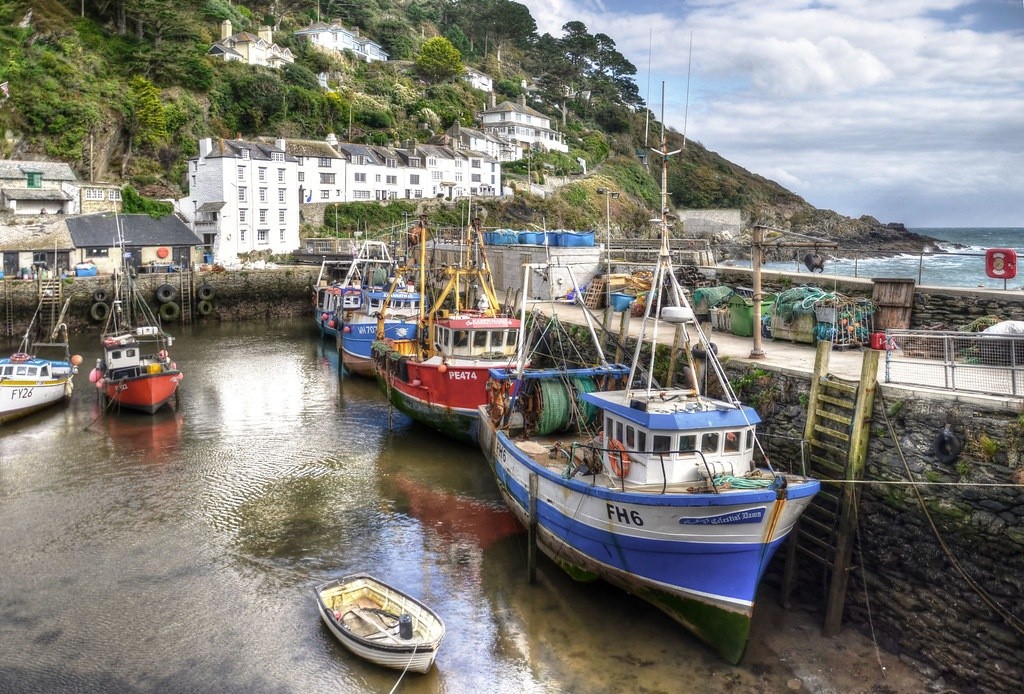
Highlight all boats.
[292,236,359,264]
[478,29,824,667]
[309,256,364,337]
[90,190,183,416]
[370,213,533,440]
[0,238,83,429]
[312,571,446,674]
[332,239,428,378]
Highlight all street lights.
[334,203,339,236]
[400,211,409,261]
[596,187,619,306]
[474,217,480,293]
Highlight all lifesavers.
[93,289,108,302]
[198,301,213,315]
[159,302,180,321]
[104,338,118,344]
[156,284,177,303]
[608,438,629,478]
[197,284,216,301]
[90,302,110,321]
[12,353,30,361]
